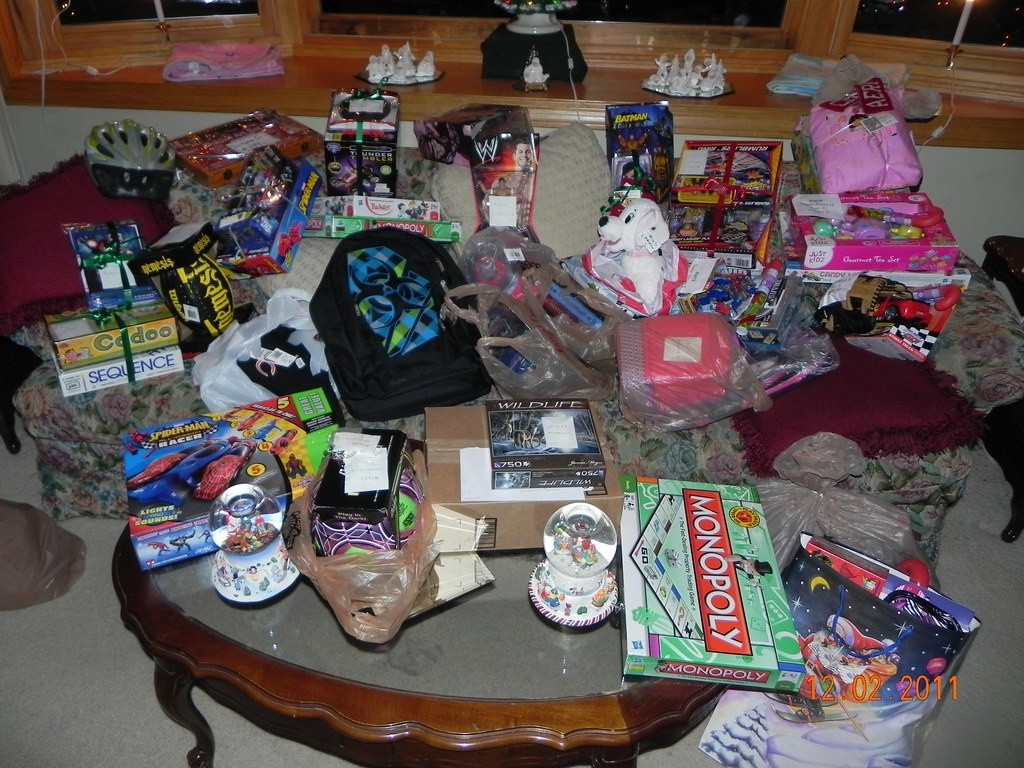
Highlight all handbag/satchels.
[613,312,841,432]
[280,450,438,643]
[698,531,983,768]
[441,226,633,400]
[190,288,341,413]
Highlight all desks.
[110,383,732,768]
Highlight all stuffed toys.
[597,198,667,314]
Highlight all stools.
[0,147,172,454]
[967,233,1024,540]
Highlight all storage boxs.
[121,388,357,568]
[306,92,461,243]
[39,284,180,369]
[420,401,627,552]
[169,108,320,190]
[141,222,216,273]
[616,473,808,691]
[214,159,320,274]
[49,343,187,397]
[600,100,971,336]
[314,426,406,525]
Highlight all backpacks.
[311,223,493,419]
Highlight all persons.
[398,45,435,76]
[525,58,543,81]
[366,44,394,79]
[513,140,534,171]
[653,49,725,91]
[478,177,522,224]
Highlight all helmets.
[87,117,177,197]
